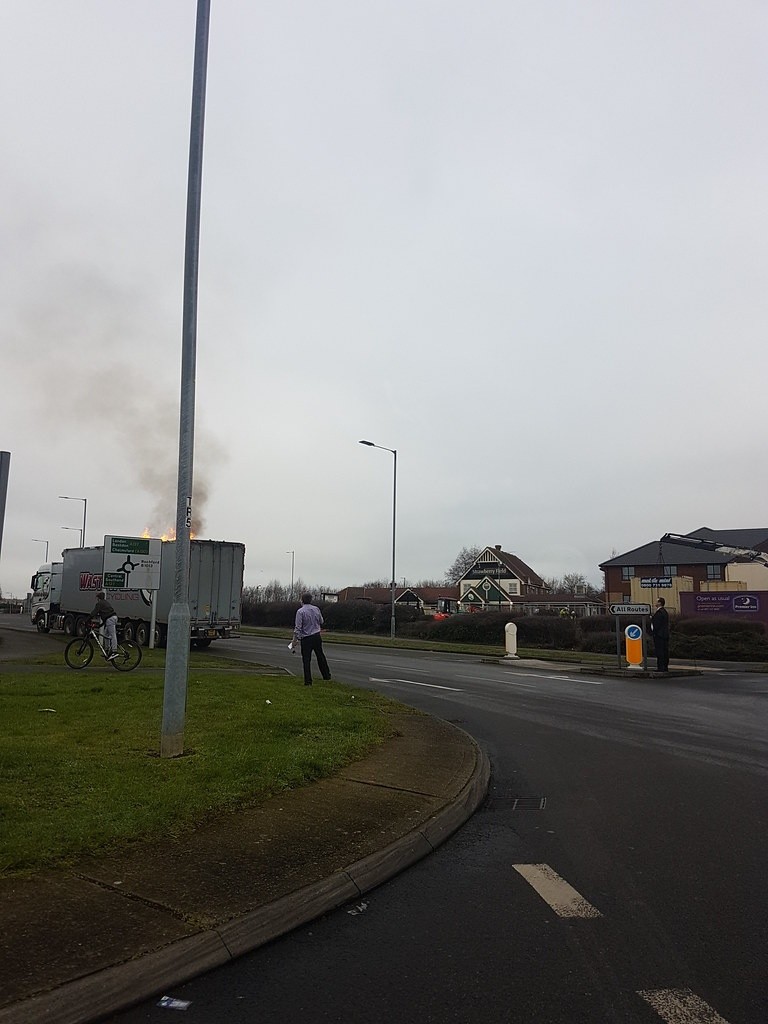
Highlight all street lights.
[359,440,398,639]
[34,540,49,563]
[58,496,87,547]
[286,551,294,603]
[61,527,82,548]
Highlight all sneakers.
[107,652,119,661]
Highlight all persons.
[649,596,669,672]
[290,594,331,686]
[91,592,119,661]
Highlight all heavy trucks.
[29,539,246,650]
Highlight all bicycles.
[64,622,143,672]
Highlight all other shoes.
[653,668,668,672]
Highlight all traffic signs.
[609,603,652,615]
